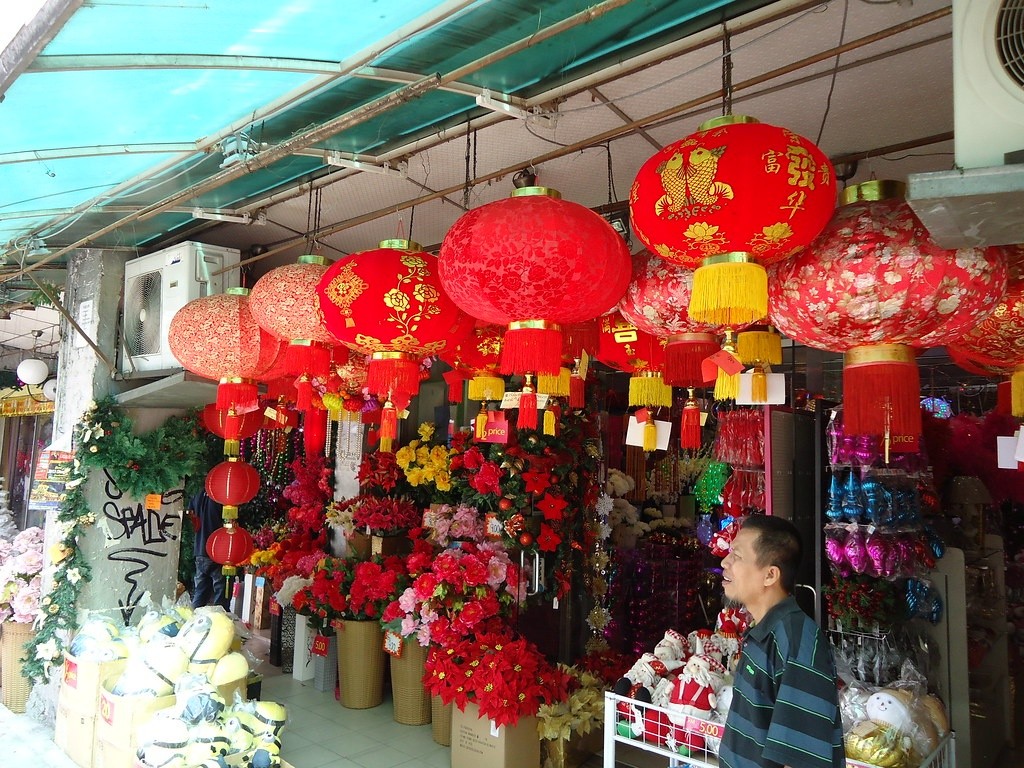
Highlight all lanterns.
[438,187,633,431]
[168,286,296,461]
[198,404,263,600]
[630,118,838,401]
[439,317,587,443]
[589,245,784,454]
[303,240,475,452]
[763,178,1005,435]
[247,251,333,415]
[948,243,1024,420]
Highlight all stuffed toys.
[65,604,287,768]
[837,679,949,768]
[618,604,754,756]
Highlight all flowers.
[0,526,46,623]
[237,420,615,739]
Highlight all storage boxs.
[92,657,247,768]
[55,649,110,768]
[542,725,603,768]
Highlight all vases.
[1,621,41,713]
[334,618,384,710]
[430,689,452,746]
[453,698,539,768]
[390,633,433,726]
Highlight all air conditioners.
[121,240,241,381]
[953,1,1024,169]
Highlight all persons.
[719,514,846,768]
[190,486,235,610]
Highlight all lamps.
[27,233,51,258]
[17,330,59,401]
[217,135,255,169]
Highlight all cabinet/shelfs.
[903,531,1011,767]
